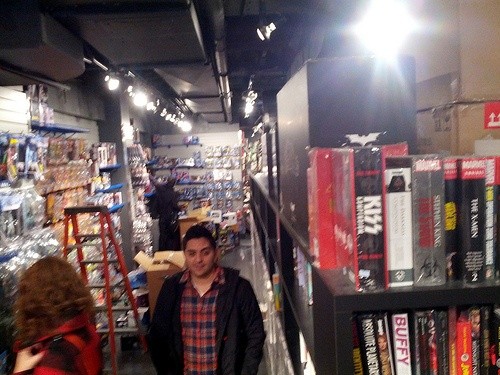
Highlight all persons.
[13,255,103,375]
[145,223,267,375]
[157,177,185,252]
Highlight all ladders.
[62,205,148,375]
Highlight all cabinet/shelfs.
[246,170,500,375]
[0,119,206,346]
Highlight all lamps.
[160,106,193,132]
[102,70,120,91]
[127,80,148,108]
[244,80,258,119]
[145,96,158,112]
[255,20,278,41]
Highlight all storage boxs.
[134,250,187,318]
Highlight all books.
[306,142,499,375]
